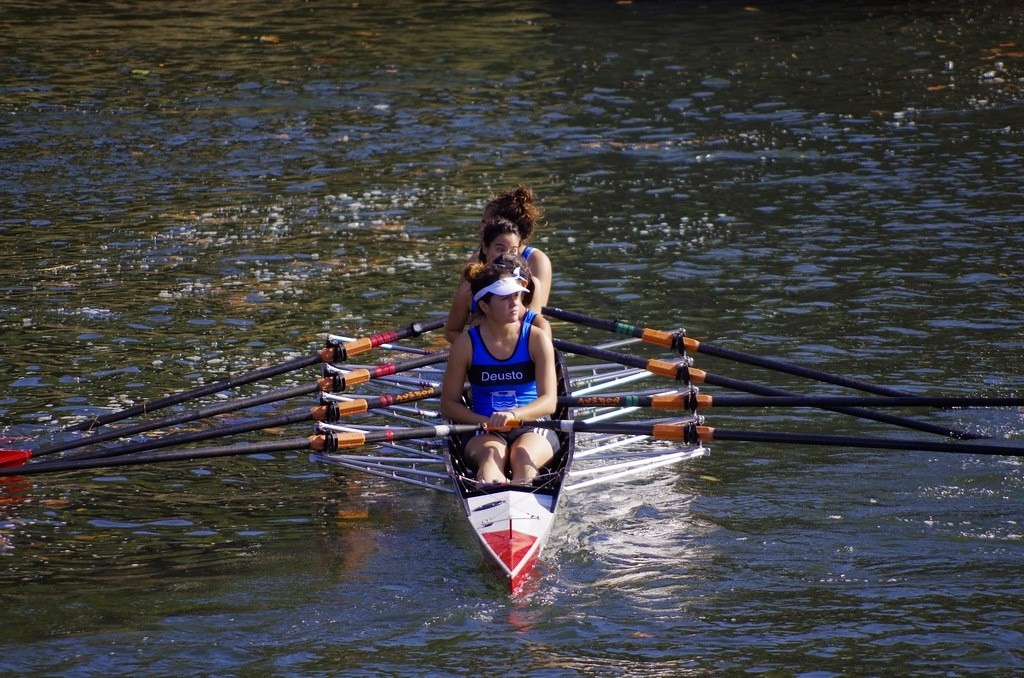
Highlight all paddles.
[0,422,486,478]
[552,334,989,439]
[504,419,1024,461]
[76,385,440,461]
[1,350,448,466]
[541,304,899,395]
[559,392,1021,411]
[67,316,448,432]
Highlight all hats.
[474,277,531,304]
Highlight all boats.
[441,334,575,596]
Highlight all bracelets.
[509,410,518,419]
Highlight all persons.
[435,186,562,487]
